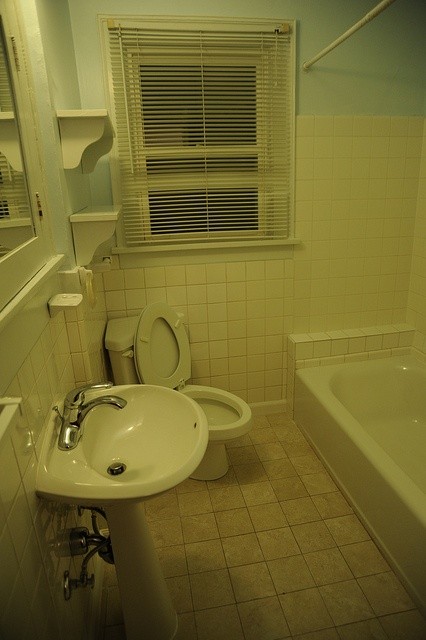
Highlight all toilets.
[105,302,252,480]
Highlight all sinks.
[81,385,200,484]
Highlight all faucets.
[52,382,127,451]
